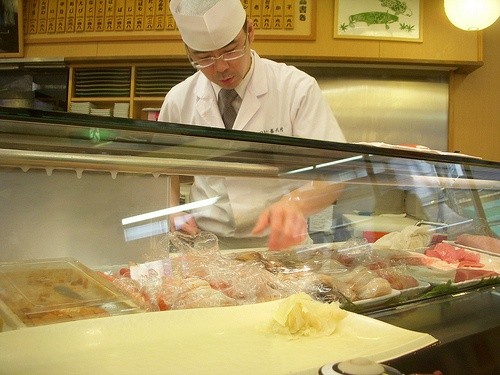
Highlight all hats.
[170,0,246,51]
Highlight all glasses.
[185,17,248,69]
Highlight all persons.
[154,0,350,248]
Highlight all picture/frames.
[333,0,423,43]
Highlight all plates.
[168,239,500,307]
[70,102,128,118]
[0,294,437,375]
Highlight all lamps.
[443,0,500,32]
